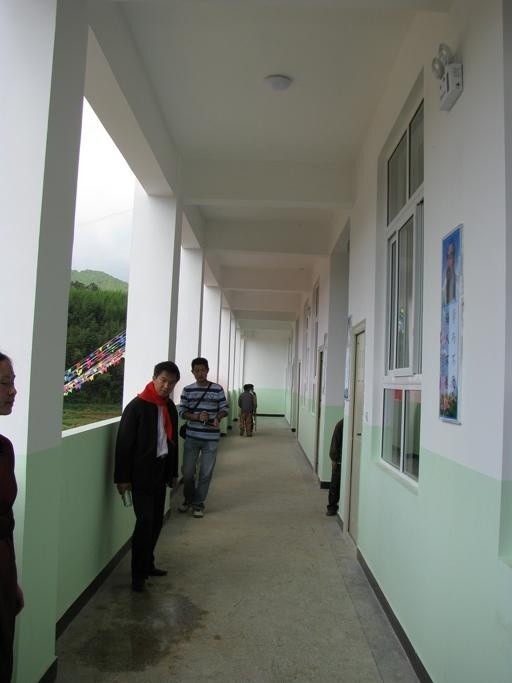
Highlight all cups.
[199,410,208,426]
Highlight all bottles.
[120,486,131,507]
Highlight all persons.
[326,418,344,516]
[246,384,257,431]
[113,361,180,593]
[0,353,25,683]
[238,384,253,437]
[178,357,230,517]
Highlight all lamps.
[261,73,293,94]
[431,42,464,111]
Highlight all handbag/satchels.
[179,421,186,439]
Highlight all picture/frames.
[439,223,464,426]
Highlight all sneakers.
[192,504,205,519]
[179,499,191,511]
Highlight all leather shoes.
[147,568,167,576]
[131,578,144,592]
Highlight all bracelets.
[197,413,200,422]
